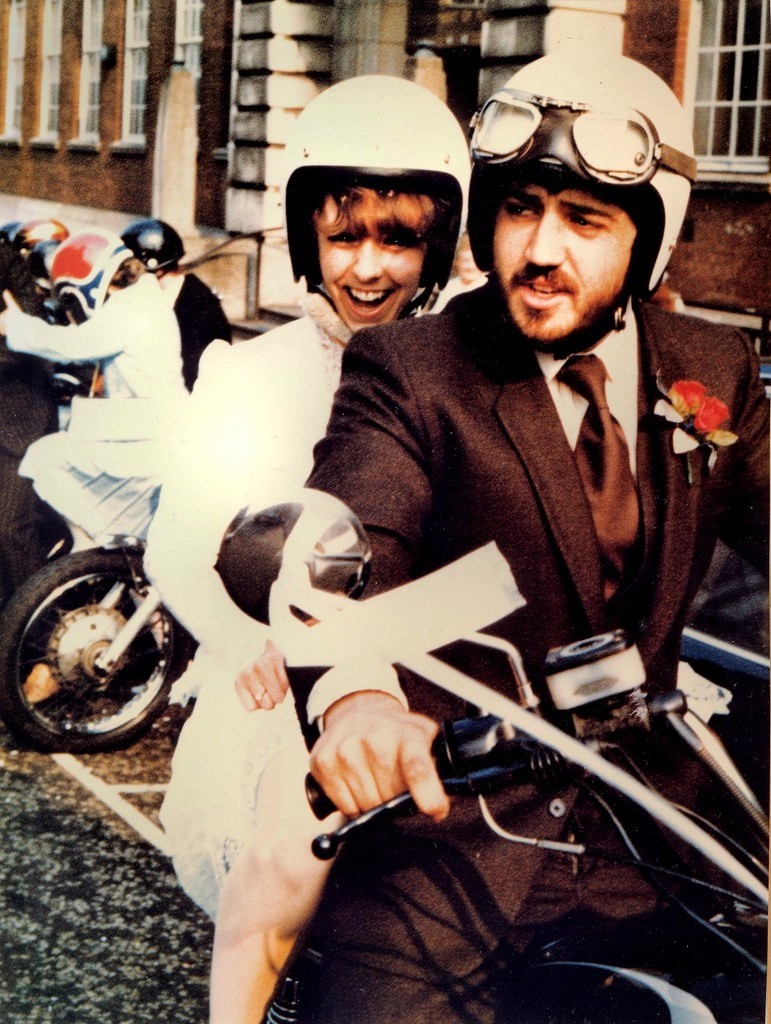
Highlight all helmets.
[0,218,71,292]
[48,232,136,321]
[120,218,186,274]
[464,43,697,303]
[279,74,473,315]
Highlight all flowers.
[653,377,742,481]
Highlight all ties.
[553,353,647,603]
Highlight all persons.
[139,71,493,1020]
[94,215,236,398]
[0,218,76,597]
[263,43,765,1021]
[2,227,191,553]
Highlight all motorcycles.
[163,473,767,1024]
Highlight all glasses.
[467,86,696,188]
